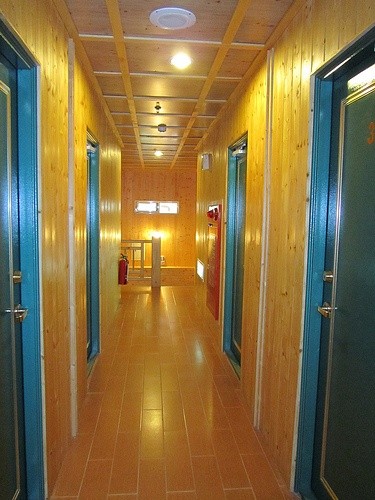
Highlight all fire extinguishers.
[118,253,129,285]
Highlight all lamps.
[159,124,167,133]
[169,55,193,69]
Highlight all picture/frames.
[131,243,146,261]
